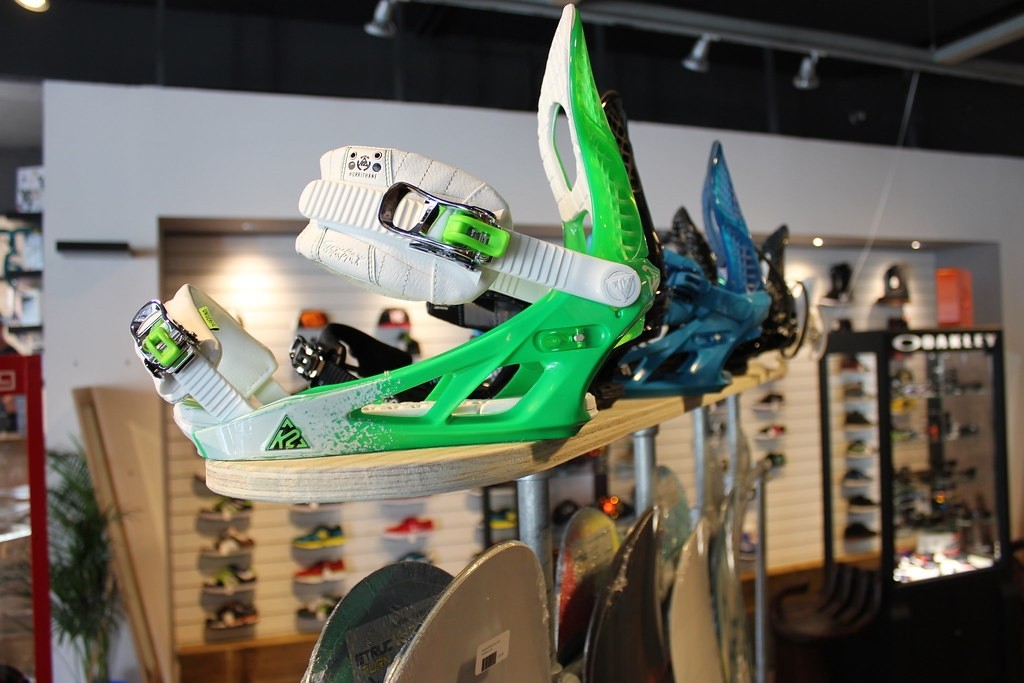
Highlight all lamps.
[363,0,398,38]
[793,50,826,88]
[684,33,721,72]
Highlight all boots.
[819,264,853,307]
[882,265,909,306]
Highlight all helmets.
[298,310,329,330]
[378,308,411,329]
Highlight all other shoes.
[471,486,516,496]
[828,316,999,577]
[758,453,785,468]
[753,394,784,411]
[0,395,20,442]
[755,425,786,440]
[287,501,341,513]
[479,509,518,529]
[384,495,431,505]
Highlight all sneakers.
[386,517,435,539]
[207,602,257,629]
[298,596,343,623]
[397,552,436,567]
[295,559,347,584]
[205,566,256,596]
[200,496,254,523]
[293,524,346,549]
[202,527,254,558]
